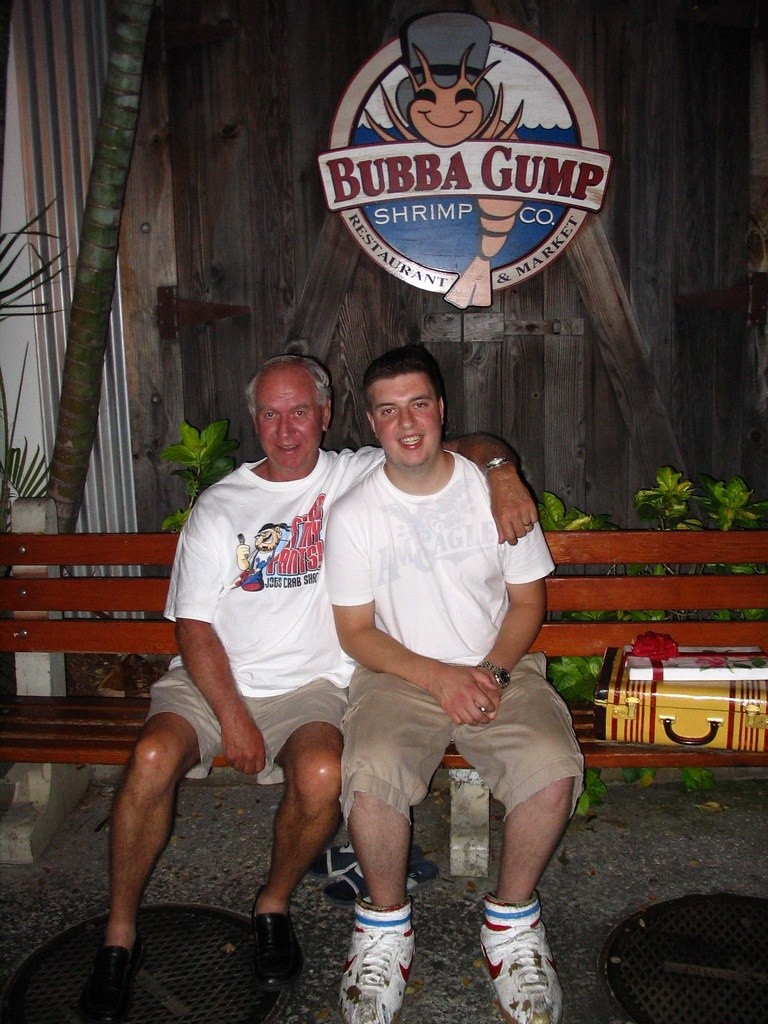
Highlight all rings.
[523,521,534,527]
[479,705,487,714]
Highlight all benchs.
[1,526,768,768]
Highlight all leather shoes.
[252,885,303,992]
[79,927,146,1024]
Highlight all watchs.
[476,661,510,690]
[484,457,515,478]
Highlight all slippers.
[310,842,439,906]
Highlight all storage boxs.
[592,643,767,754]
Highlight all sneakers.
[339,893,413,1023]
[479,889,563,1024]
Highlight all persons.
[85,355,539,1024]
[328,348,585,1024]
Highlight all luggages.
[592,647,767,754]
